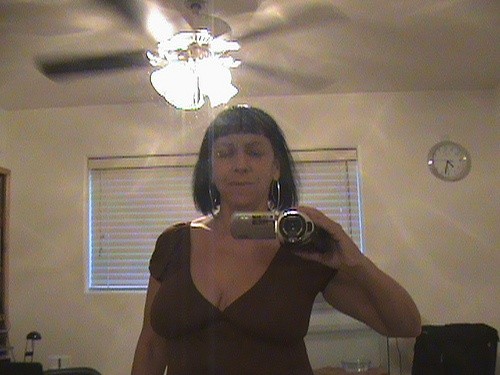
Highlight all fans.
[32,0,349,95]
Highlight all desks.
[313,364,386,375]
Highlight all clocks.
[427,139,472,181]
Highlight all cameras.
[229,209,318,250]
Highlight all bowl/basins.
[341,357,371,374]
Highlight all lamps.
[145,27,242,112]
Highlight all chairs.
[410,323,500,375]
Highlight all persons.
[130,105,421,375]
